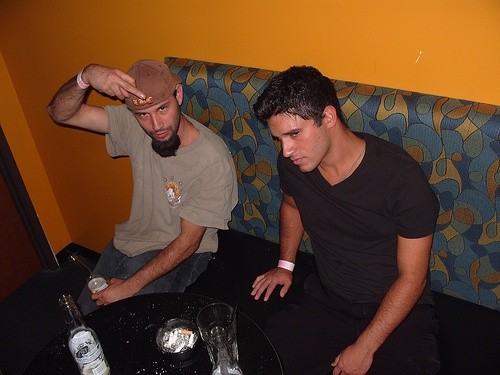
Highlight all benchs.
[162,55,500,375]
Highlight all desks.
[32,292,283,375]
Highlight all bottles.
[59,294,111,374]
[68,255,108,305]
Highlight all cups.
[195,302,243,375]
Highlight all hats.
[125,59,183,109]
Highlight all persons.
[251,64,439,375]
[48,58,239,317]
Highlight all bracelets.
[277,259,295,272]
[76,69,89,90]
